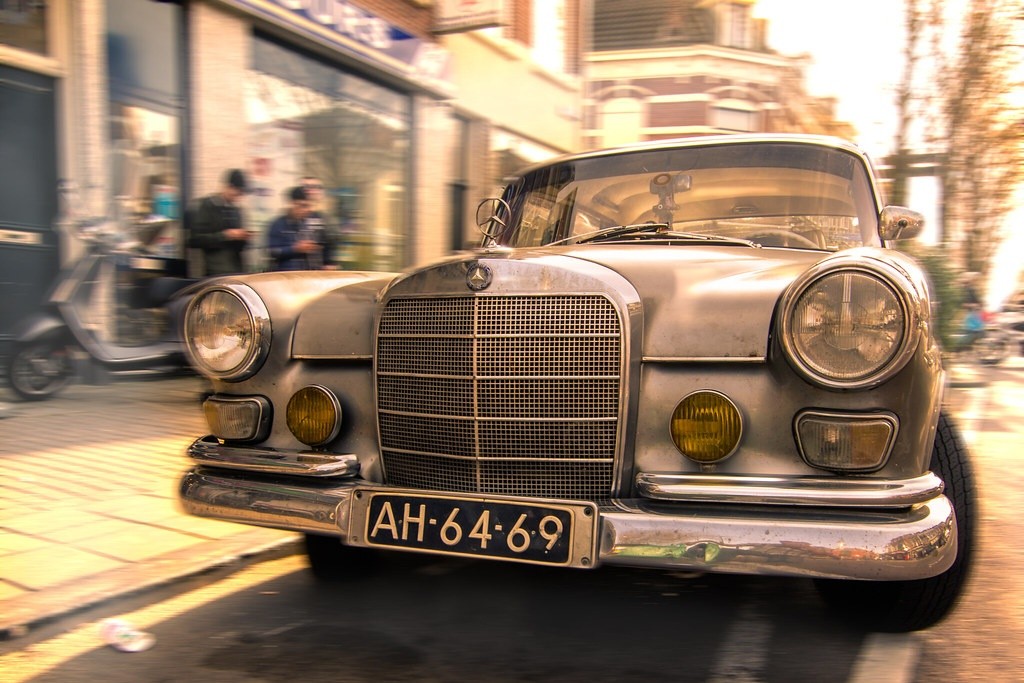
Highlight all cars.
[174,134,982,637]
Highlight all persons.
[263,176,344,271]
[186,167,254,282]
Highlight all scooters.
[2,216,223,401]
